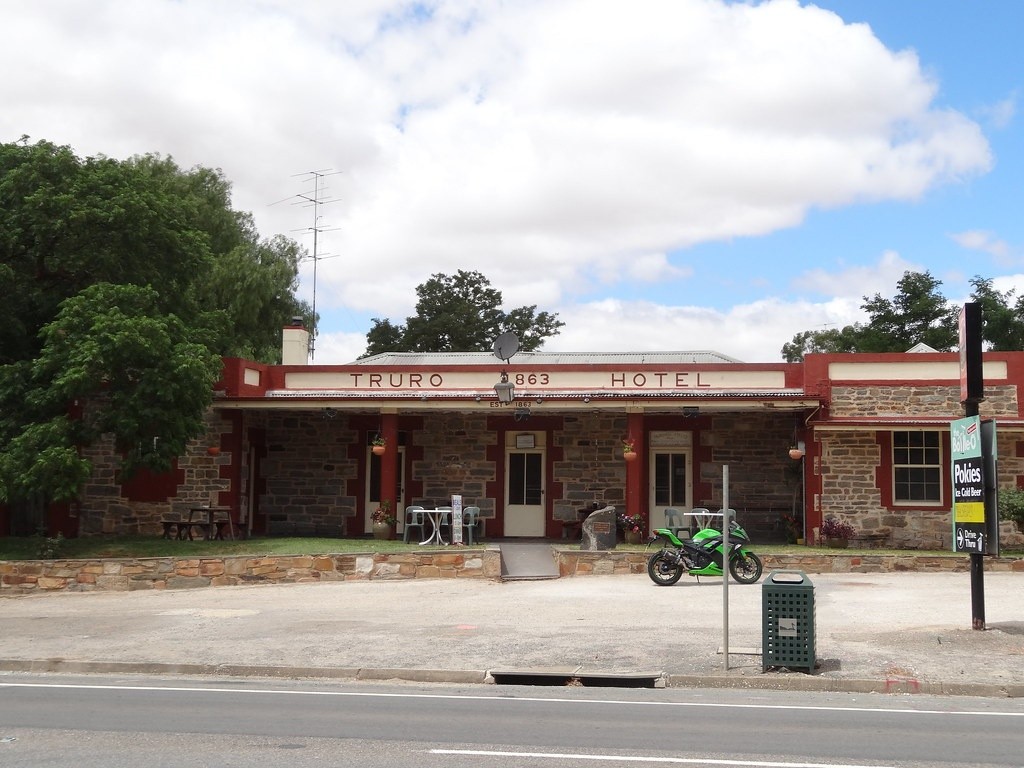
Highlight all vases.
[625,532,641,544]
[828,539,848,549]
[624,452,637,460]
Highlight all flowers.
[621,439,636,453]
[820,519,856,540]
[617,511,646,538]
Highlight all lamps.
[514,408,531,422]
[325,410,338,419]
[683,407,699,417]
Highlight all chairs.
[437,507,452,542]
[689,508,711,532]
[402,506,425,544]
[716,509,736,528]
[462,507,481,545]
[664,509,689,547]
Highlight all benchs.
[160,520,248,541]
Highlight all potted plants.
[207,439,221,455]
[369,498,400,540]
[788,445,803,458]
[371,437,386,456]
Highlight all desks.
[183,506,232,541]
[412,509,452,545]
[683,513,723,531]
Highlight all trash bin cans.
[0,503,9,536]
[47,498,80,539]
[761,570,817,675]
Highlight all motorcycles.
[648,520,763,586]
